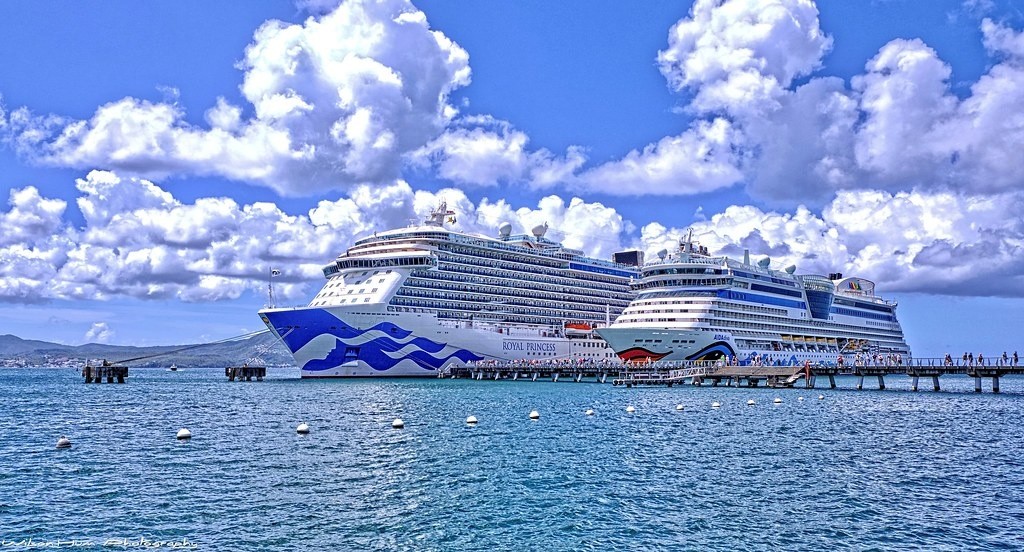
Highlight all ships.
[257,201,637,379]
[594,228,913,367]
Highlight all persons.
[962,352,967,366]
[1003,351,1008,367]
[969,352,973,366]
[1013,350,1019,366]
[978,353,983,366]
[943,353,954,367]
[466,349,905,369]
